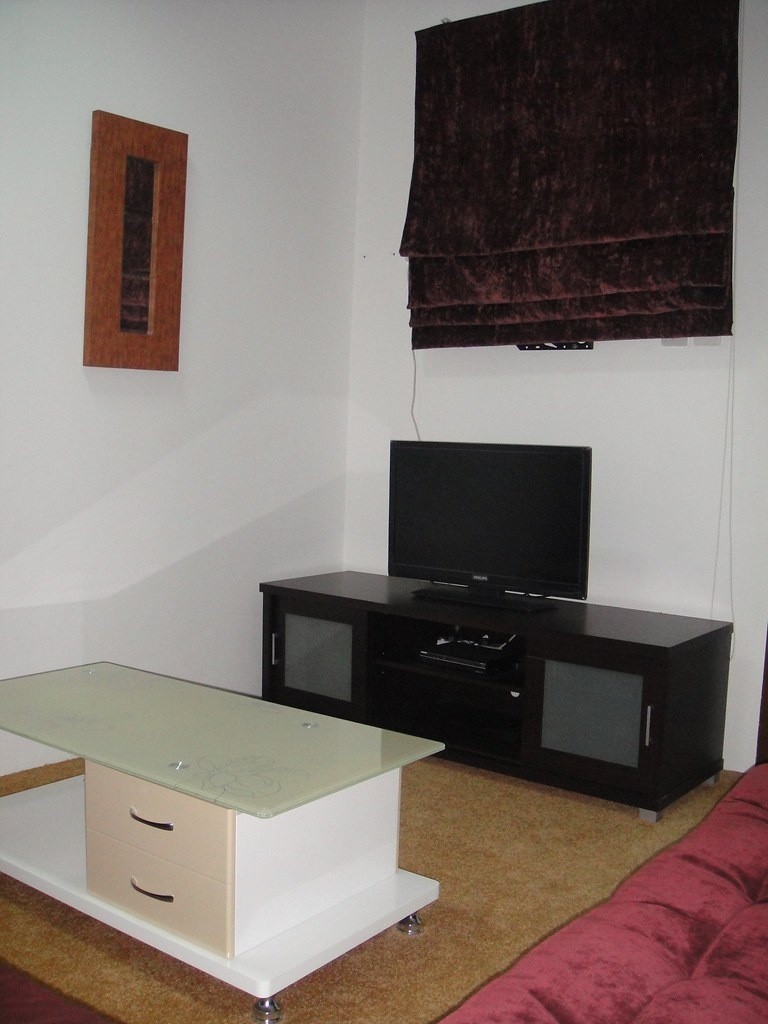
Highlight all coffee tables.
[0,660,445,1024]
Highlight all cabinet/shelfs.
[259,571,734,822]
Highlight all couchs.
[433,764,768,1024]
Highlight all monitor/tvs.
[389,441,593,614]
